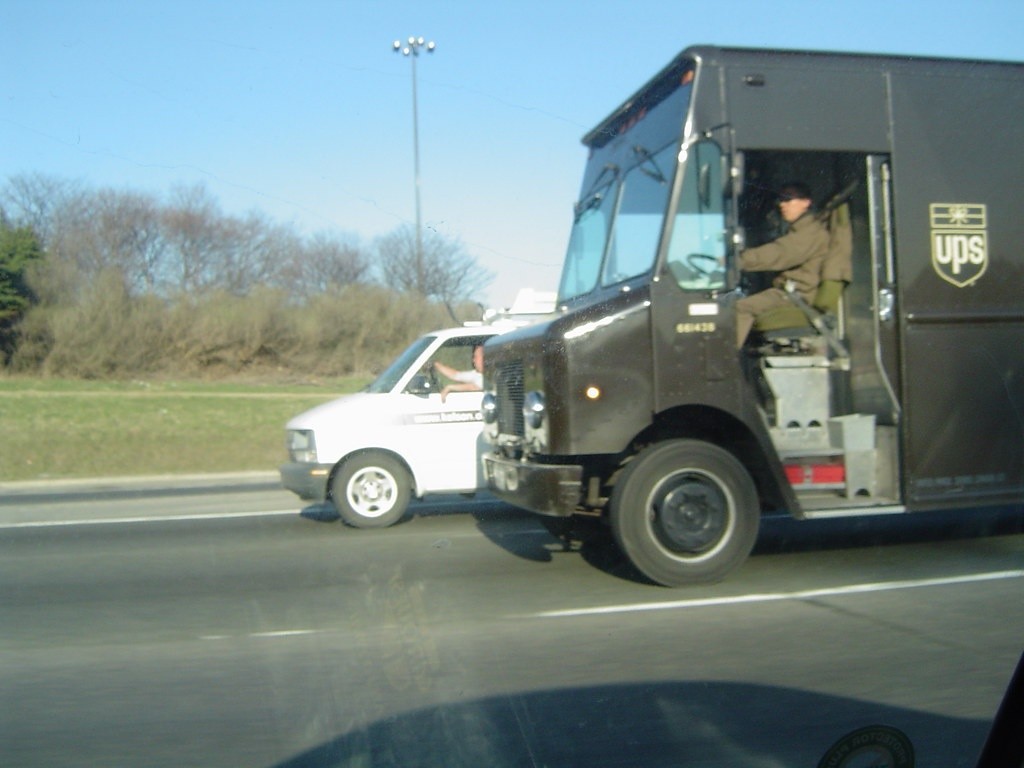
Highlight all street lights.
[392,35,437,300]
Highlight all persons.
[422,346,483,402]
[736,181,830,354]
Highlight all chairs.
[757,200,853,346]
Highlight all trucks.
[477,45,1024,590]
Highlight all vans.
[277,306,561,528]
